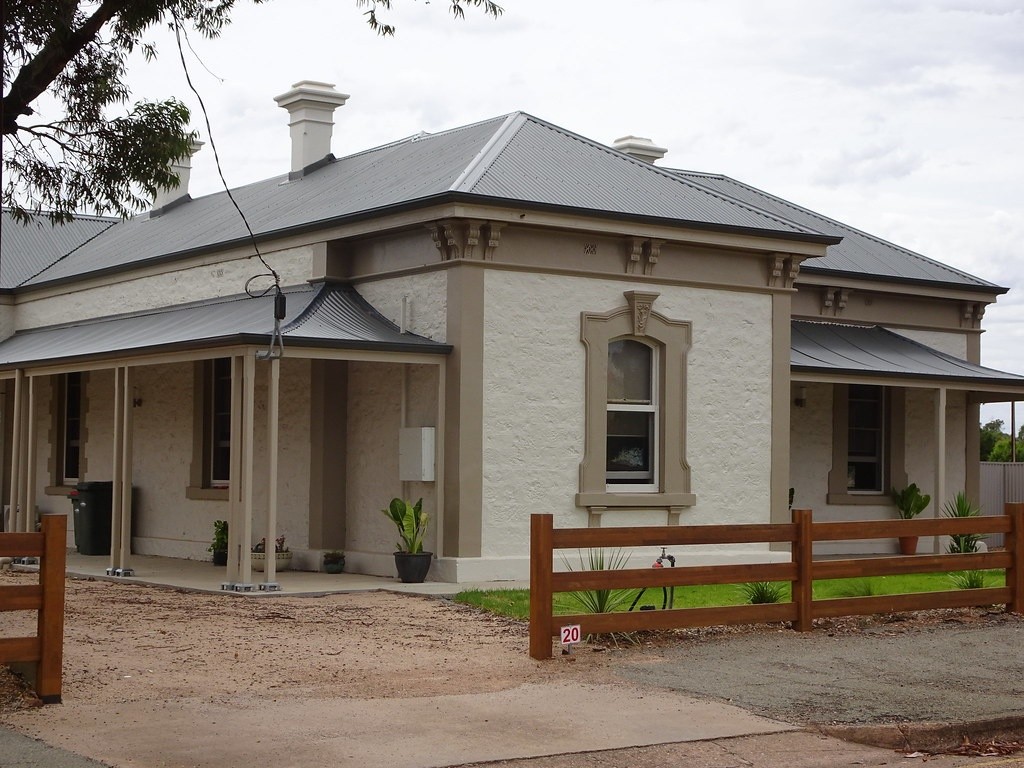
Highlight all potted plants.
[205,519,228,566]
[380,497,434,583]
[323,550,345,575]
[891,483,931,556]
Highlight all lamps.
[795,387,806,408]
[133,387,142,407]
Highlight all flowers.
[249,535,290,554]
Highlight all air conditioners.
[4,505,39,532]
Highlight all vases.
[250,552,292,572]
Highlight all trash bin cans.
[67,481,113,556]
